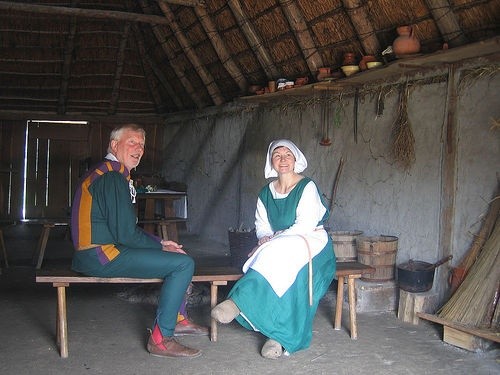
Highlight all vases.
[342,52,357,66]
[392,25,421,59]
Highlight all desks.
[136,191,185,243]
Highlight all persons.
[211,139,335,358]
[71,122,210,358]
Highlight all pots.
[397,259,435,293]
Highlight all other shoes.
[174,319,211,337]
[147,328,202,358]
[210,300,241,324]
[260,339,282,360]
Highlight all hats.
[263,139,308,178]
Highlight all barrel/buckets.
[355,235,399,282]
[327,231,364,263]
[228,231,258,267]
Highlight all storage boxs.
[170,181,187,192]
[142,175,155,187]
[319,66,332,77]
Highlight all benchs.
[17,215,189,270]
[0,219,15,269]
[33,260,376,361]
